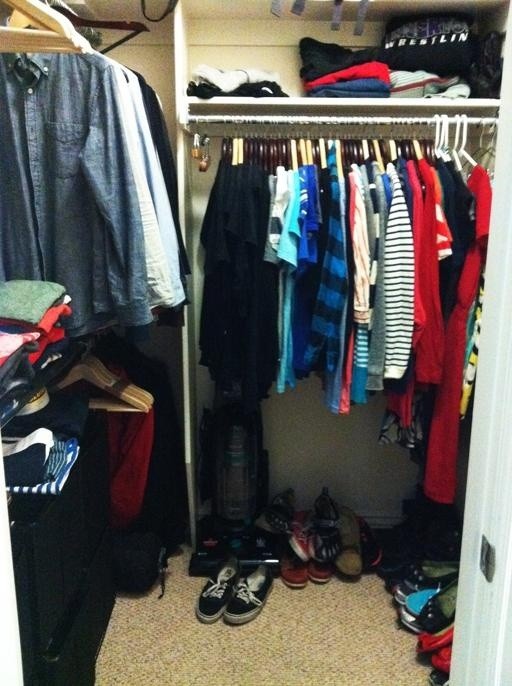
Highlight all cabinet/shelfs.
[1,409,123,686]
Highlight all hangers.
[41,352,158,416]
[187,112,504,190]
[0,0,152,64]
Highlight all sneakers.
[386,527,463,686]
[255,487,385,587]
[224,563,273,625]
[196,553,240,623]
